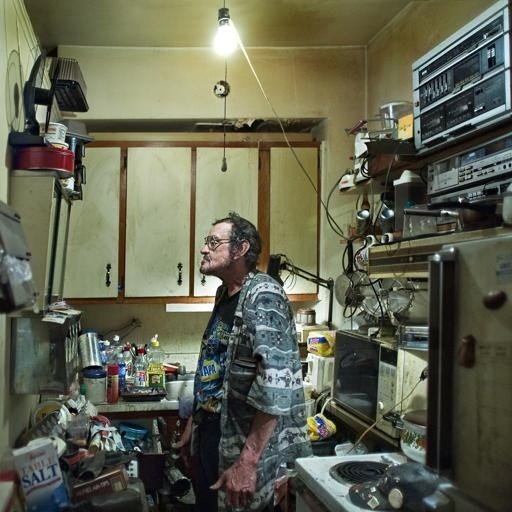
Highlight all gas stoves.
[292,448,487,512]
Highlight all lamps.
[265,250,334,330]
[211,0,239,58]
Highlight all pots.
[401,191,512,231]
[380,407,427,466]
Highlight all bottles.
[106,365,118,404]
[101,333,168,386]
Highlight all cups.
[378,101,413,131]
[355,235,379,266]
[167,377,195,400]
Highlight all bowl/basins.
[39,121,68,142]
[120,422,147,441]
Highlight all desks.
[90,399,199,482]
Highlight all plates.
[119,385,167,402]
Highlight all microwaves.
[330,328,430,443]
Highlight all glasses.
[203,235,238,251]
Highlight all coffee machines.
[305,353,335,394]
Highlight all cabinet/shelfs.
[8,169,73,312]
[54,139,125,303]
[337,109,511,251]
[264,139,318,300]
[125,140,263,303]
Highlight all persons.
[172,209,314,512]
